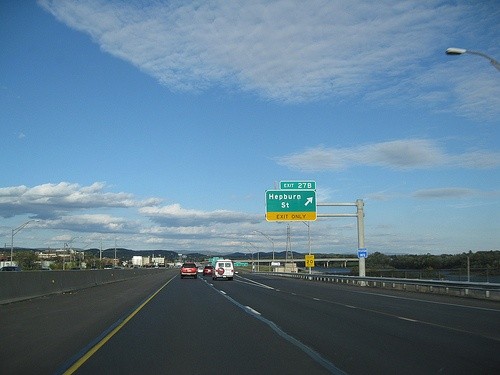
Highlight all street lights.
[98,240,104,269]
[62,236,82,269]
[10,219,40,271]
[114,236,119,269]
[246,241,259,272]
[251,230,275,272]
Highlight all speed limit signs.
[304,254,315,268]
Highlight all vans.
[212,259,234,281]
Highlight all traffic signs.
[265,180,317,222]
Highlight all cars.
[203,265,215,276]
[104,264,113,269]
[194,263,204,274]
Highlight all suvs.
[180,261,198,279]
